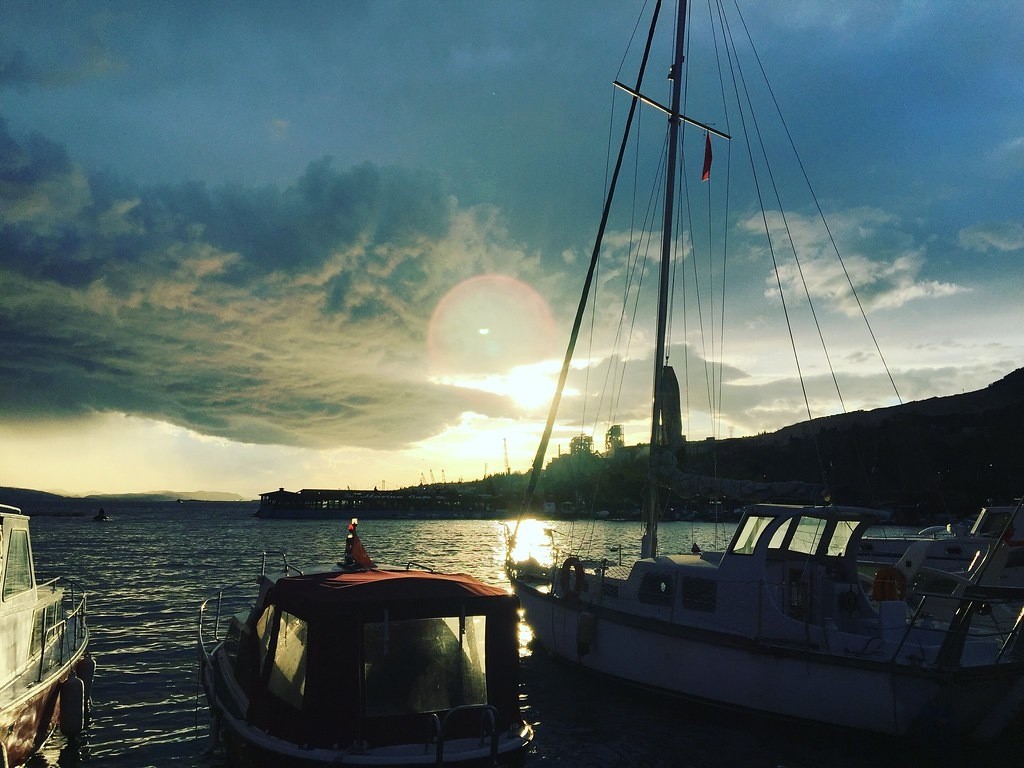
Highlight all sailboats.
[504,0,1024,746]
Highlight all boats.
[787,495,1024,630]
[0,502,91,768]
[195,514,547,768]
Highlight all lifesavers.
[871,566,907,604]
[558,559,586,600]
